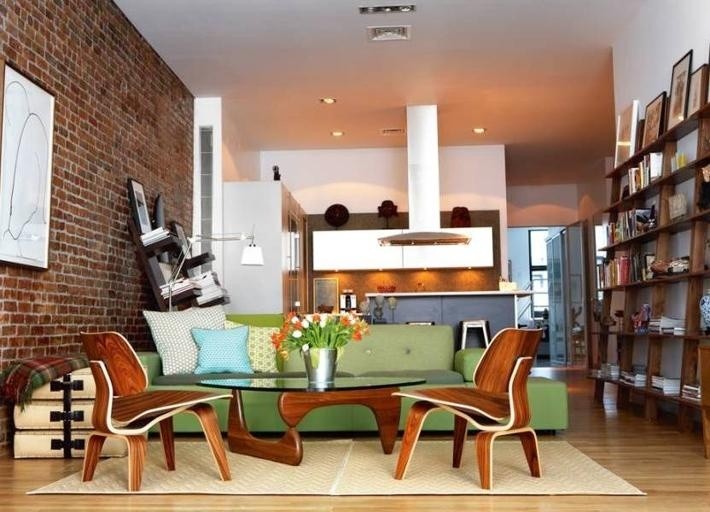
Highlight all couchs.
[134,324,567,442]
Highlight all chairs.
[79,329,238,491]
[388,326,547,488]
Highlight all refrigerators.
[222,181,300,313]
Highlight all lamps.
[167,228,262,313]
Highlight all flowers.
[270,308,370,361]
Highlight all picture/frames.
[1,62,57,271]
[126,176,154,236]
[613,48,708,169]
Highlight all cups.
[499,282,517,292]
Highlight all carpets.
[26,436,644,499]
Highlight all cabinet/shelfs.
[586,100,709,456]
[127,218,232,309]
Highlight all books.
[648,316,687,338]
[590,363,702,403]
[137,224,230,306]
[600,150,663,289]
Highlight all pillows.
[142,300,285,376]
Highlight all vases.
[299,346,337,382]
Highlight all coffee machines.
[340,294,357,314]
